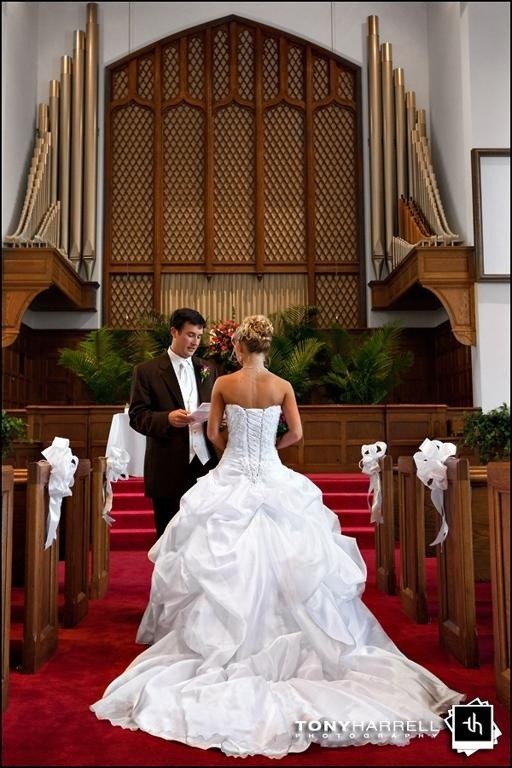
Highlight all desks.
[105,413,146,477]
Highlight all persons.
[128,306,224,542]
[182,312,306,660]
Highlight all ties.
[180,361,193,397]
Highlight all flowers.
[203,305,240,369]
[200,364,210,384]
[276,414,288,437]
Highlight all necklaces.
[242,366,269,371]
